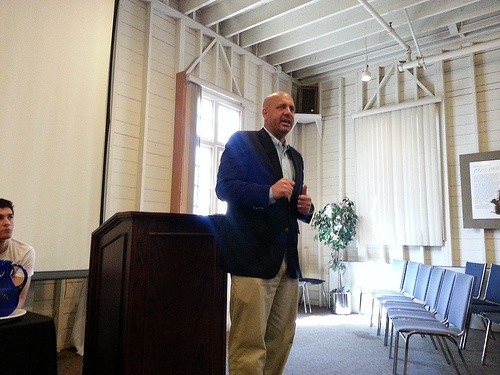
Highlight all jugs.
[0,259,28,318]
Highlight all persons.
[214,92,314,375]
[0,198,35,308]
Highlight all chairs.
[359,259,500,375]
[299,265,326,314]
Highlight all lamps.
[361,37,372,81]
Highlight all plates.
[0,309,27,319]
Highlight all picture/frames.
[459,151,500,229]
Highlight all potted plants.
[312,198,358,316]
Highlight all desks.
[0,310,57,375]
